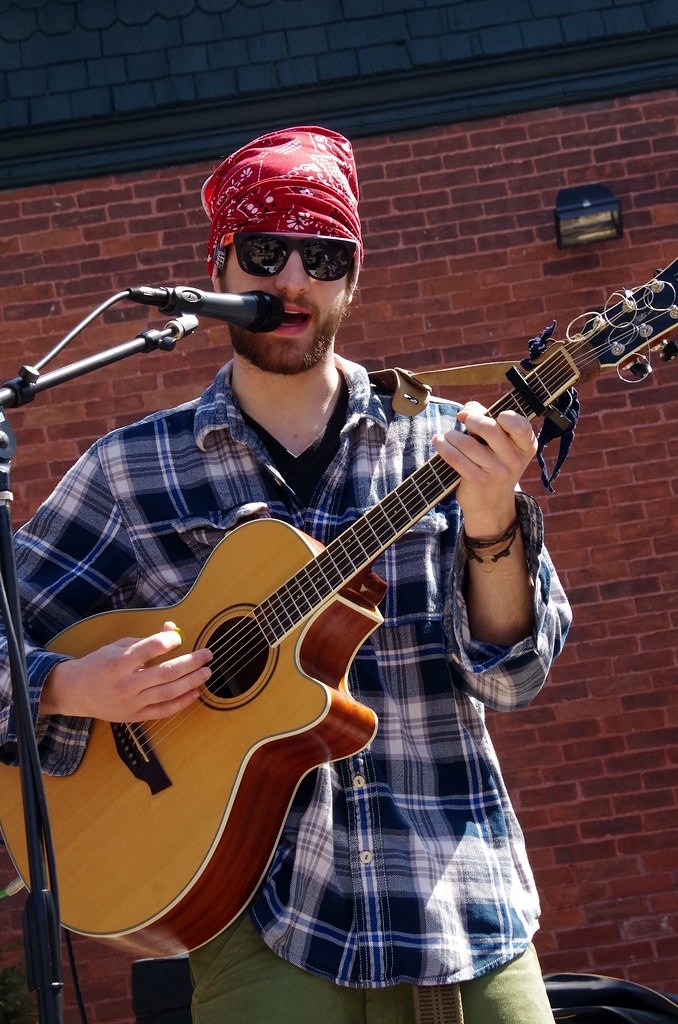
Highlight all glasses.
[222,231,356,281]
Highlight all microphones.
[125,286,285,334]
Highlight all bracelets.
[464,512,521,574]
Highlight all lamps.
[553,183,625,250]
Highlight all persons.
[0,122,573,1024]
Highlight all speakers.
[130,957,193,1024]
